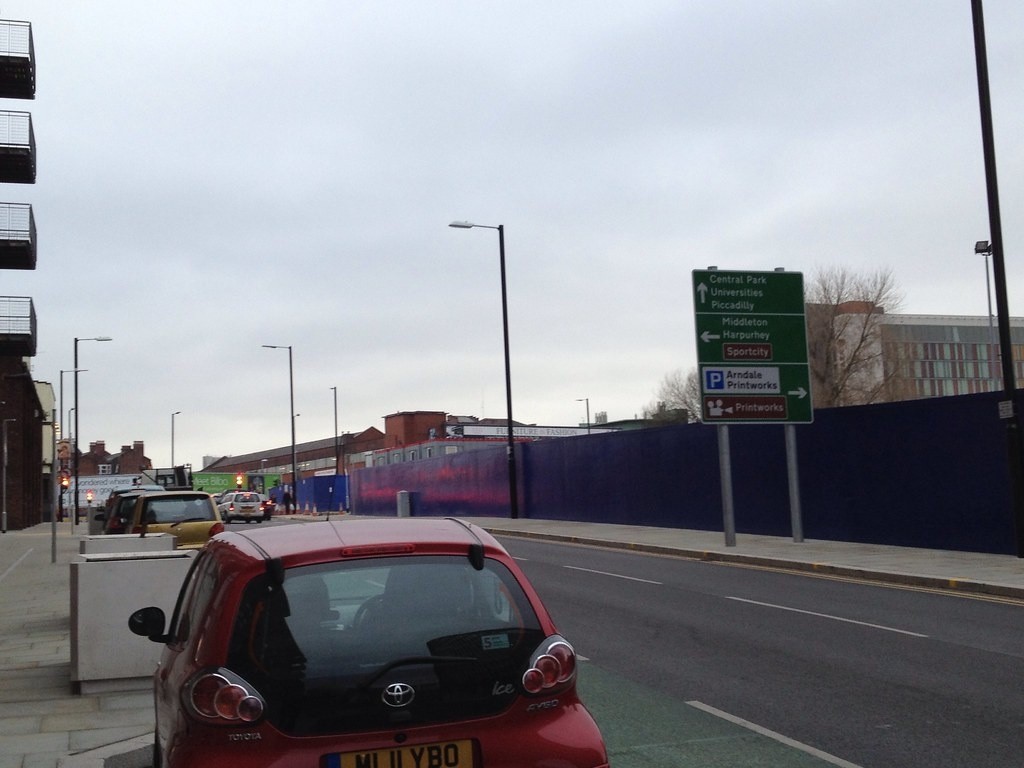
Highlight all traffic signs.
[693,313,810,363]
[691,270,808,314]
[697,364,815,426]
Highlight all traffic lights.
[86,492,93,504]
[61,479,70,489]
[236,476,244,487]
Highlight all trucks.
[131,463,196,492]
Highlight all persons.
[269,493,278,504]
[282,489,293,514]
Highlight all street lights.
[331,386,338,475]
[973,241,998,393]
[1,417,17,534]
[261,459,268,471]
[171,411,181,469]
[60,369,89,521]
[67,406,76,469]
[262,342,297,516]
[73,336,115,526]
[448,220,517,521]
[575,398,593,438]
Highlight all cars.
[94,485,167,534]
[119,490,225,556]
[209,489,274,521]
[126,515,611,768]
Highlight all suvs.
[217,491,264,525]
